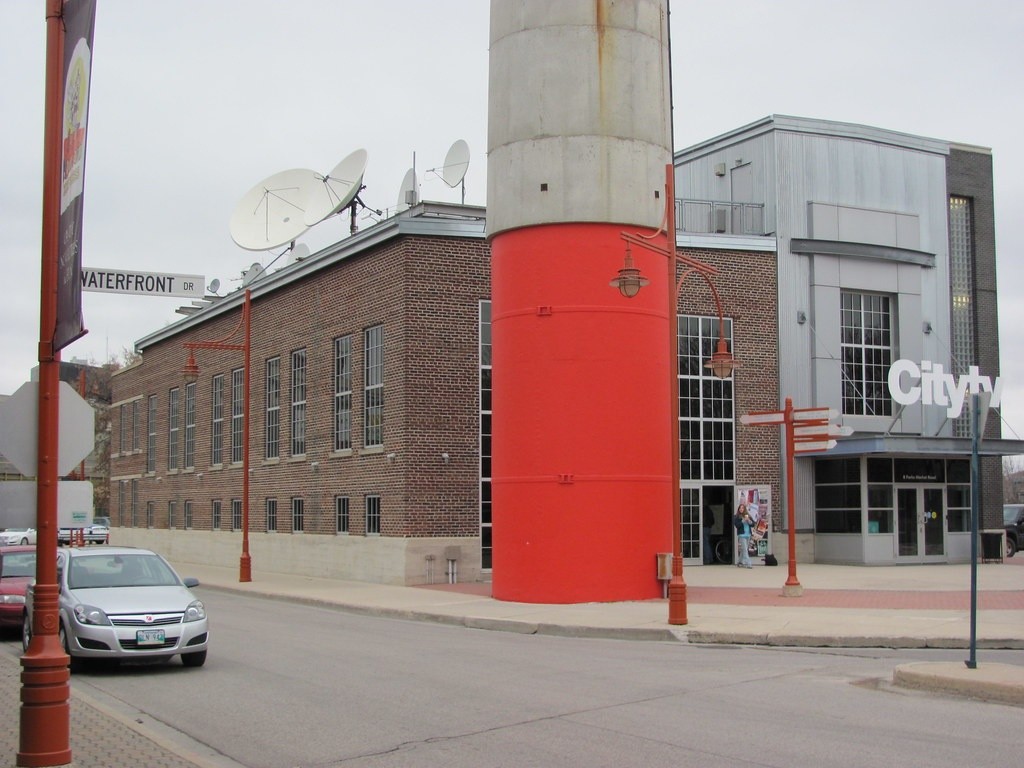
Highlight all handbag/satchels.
[765,553,777,565]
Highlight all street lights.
[608,164,737,627]
[181,290,255,584]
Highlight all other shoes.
[703,559,714,564]
[738,563,751,568]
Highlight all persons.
[734,504,755,569]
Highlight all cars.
[1003,503,1024,558]
[55,516,110,546]
[0,527,37,545]
[0,544,36,634]
[21,544,210,674]
